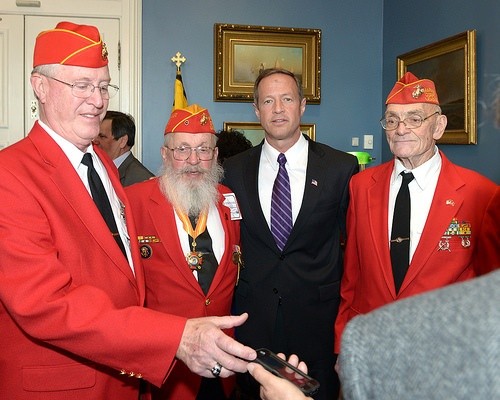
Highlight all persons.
[0,22,257,400]
[92,110,155,187]
[123,104,241,400]
[247,269,500,400]
[334,72,500,375]
[216,68,359,400]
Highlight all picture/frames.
[223,122,315,147]
[396,28,478,145]
[214,23,322,105]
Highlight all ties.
[270,154,292,250]
[188,187,219,297]
[81,153,127,262]
[389,171,414,292]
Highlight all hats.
[32,22,109,68]
[384,71,440,104]
[164,105,216,134]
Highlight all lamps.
[346,151,375,170]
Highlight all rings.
[212,362,222,376]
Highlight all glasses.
[380,111,439,131]
[32,72,119,100]
[164,145,215,162]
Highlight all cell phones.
[253,348,320,396]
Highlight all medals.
[187,251,202,270]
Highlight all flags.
[172,73,188,112]
